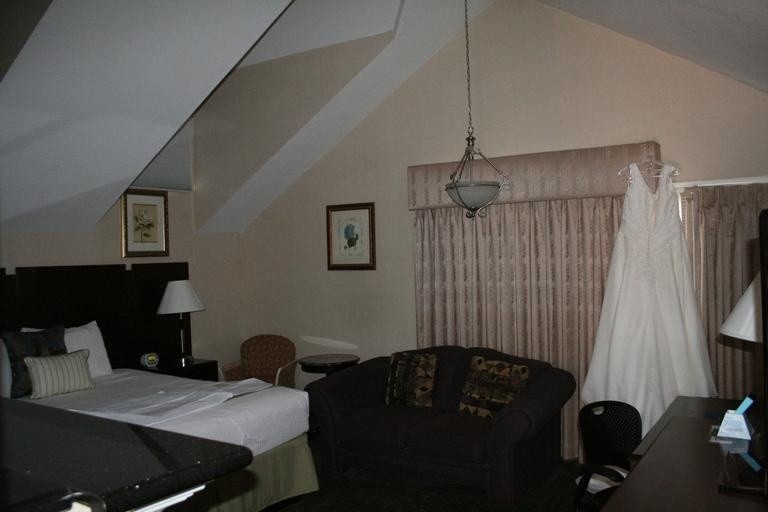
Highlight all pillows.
[384,350,437,411]
[20,321,117,386]
[1,318,67,400]
[22,349,95,400]
[1,338,13,398]
[454,356,526,423]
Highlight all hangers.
[620,161,682,184]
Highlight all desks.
[599,395,766,512]
[2,395,253,512]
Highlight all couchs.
[302,343,576,512]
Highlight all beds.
[0,261,320,510]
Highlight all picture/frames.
[122,188,170,257]
[326,201,379,270]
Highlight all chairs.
[573,401,645,504]
[222,332,299,387]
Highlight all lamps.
[156,275,208,364]
[445,2,511,218]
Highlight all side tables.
[295,353,362,387]
